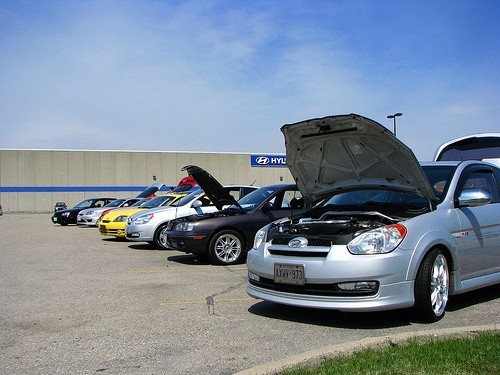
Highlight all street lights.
[387,112,402,137]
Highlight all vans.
[433,133,500,165]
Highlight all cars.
[77,182,167,227]
[124,179,290,250]
[246,113,500,322]
[98,194,213,239]
[100,176,197,220]
[0,205,4,215]
[54,202,66,214]
[163,164,307,266]
[51,197,117,226]
